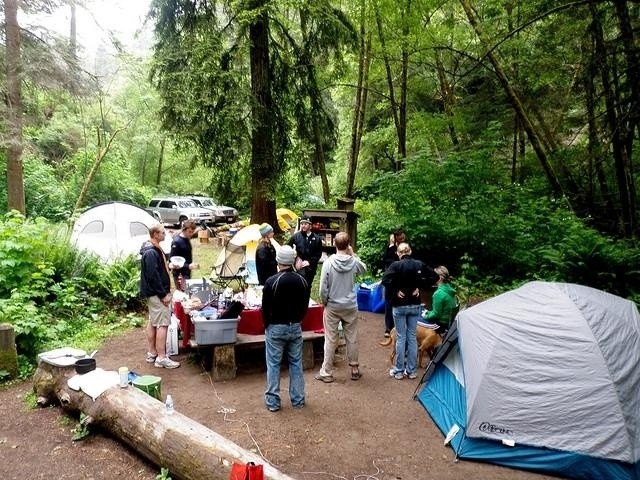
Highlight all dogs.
[377,324,444,369]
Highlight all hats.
[276,245,296,265]
[259,223,273,236]
[300,216,312,225]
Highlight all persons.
[262,245,311,413]
[385,229,411,338]
[139,222,181,368]
[172,219,196,288]
[418,265,459,334]
[255,221,278,285]
[383,242,439,381]
[315,232,367,383]
[288,217,322,287]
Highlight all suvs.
[147,199,213,229]
[191,197,236,224]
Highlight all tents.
[275,206,300,232]
[70,203,173,269]
[209,224,282,286]
[414,281,640,480]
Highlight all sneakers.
[315,371,335,383]
[390,369,404,380]
[146,352,158,363]
[352,371,363,381]
[155,356,182,369]
[404,369,419,380]
[267,403,282,413]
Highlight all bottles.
[165,393,174,415]
[119,367,128,387]
[201,285,233,312]
